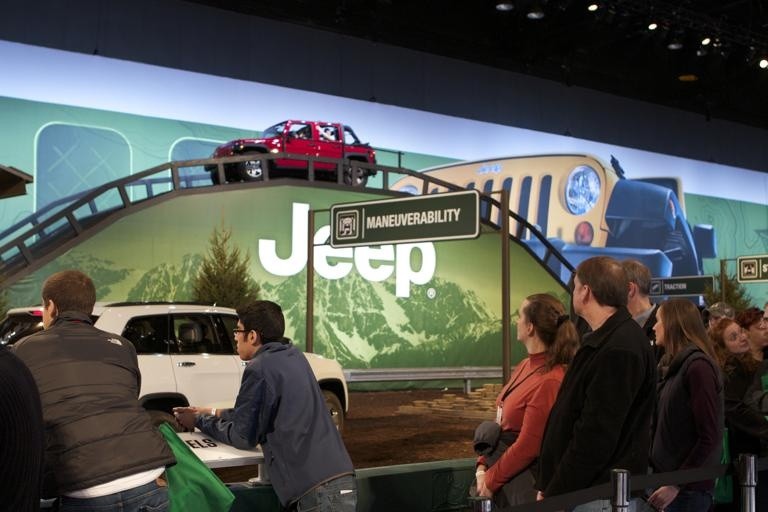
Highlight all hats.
[702,302,735,320]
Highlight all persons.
[533,255,657,512]
[11,270,178,511]
[0,346,42,512]
[475,293,581,512]
[172,300,358,512]
[703,301,768,512]
[622,259,666,359]
[645,299,727,512]
[323,128,331,140]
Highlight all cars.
[0,301,348,437]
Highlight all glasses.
[233,328,248,336]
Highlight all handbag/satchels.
[159,422,235,511]
[713,428,734,504]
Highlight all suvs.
[392,154,716,314]
[204,120,376,187]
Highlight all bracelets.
[475,471,486,476]
[211,408,216,416]
